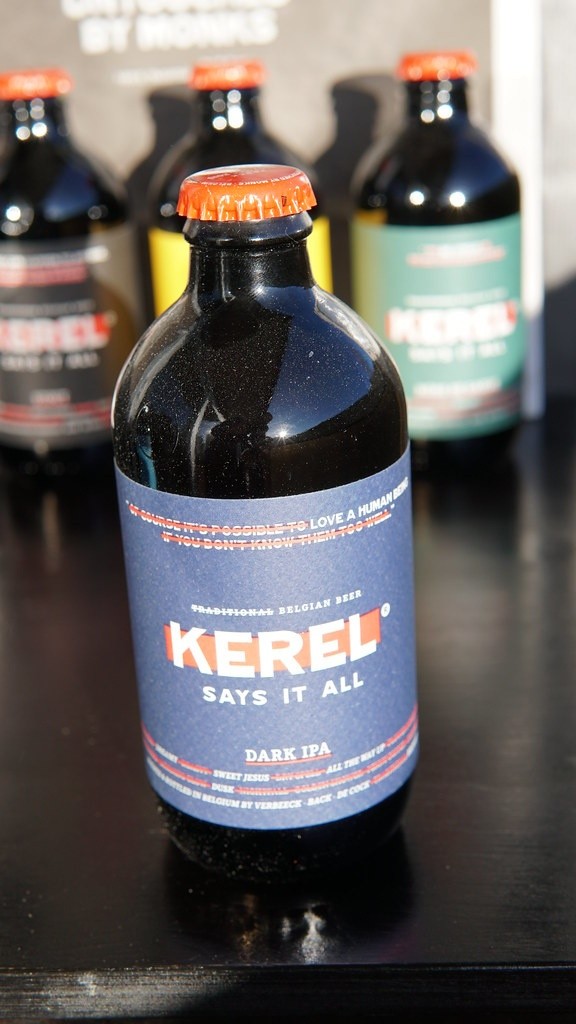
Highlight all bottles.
[351,51,521,476]
[113,162,415,954]
[142,58,334,332]
[0,70,136,532]
